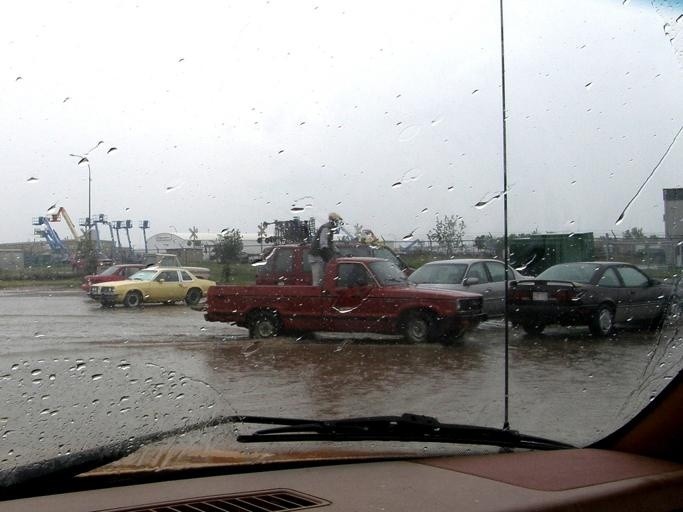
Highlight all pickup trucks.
[81,262,147,294]
[200,254,488,348]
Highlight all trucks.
[248,235,415,287]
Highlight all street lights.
[68,153,92,240]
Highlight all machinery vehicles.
[30,203,156,272]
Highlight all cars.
[152,252,212,280]
[507,259,682,338]
[403,257,534,321]
[87,266,219,309]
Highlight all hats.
[329,213,338,221]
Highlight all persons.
[308,213,340,286]
